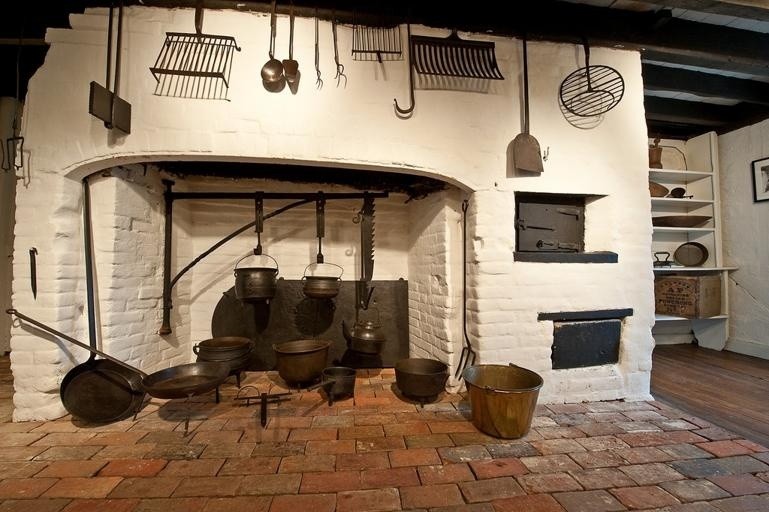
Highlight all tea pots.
[342,306,386,355]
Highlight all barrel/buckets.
[461,362,544,439]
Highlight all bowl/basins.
[674,241,708,268]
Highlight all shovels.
[283,7,298,83]
[514,41,543,173]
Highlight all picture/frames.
[751,157,769,203]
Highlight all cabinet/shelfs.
[650,128,740,353]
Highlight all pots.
[59,178,147,427]
[394,358,448,409]
[5,308,231,437]
[272,339,330,393]
[308,366,357,407]
[193,337,256,389]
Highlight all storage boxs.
[653,274,720,320]
